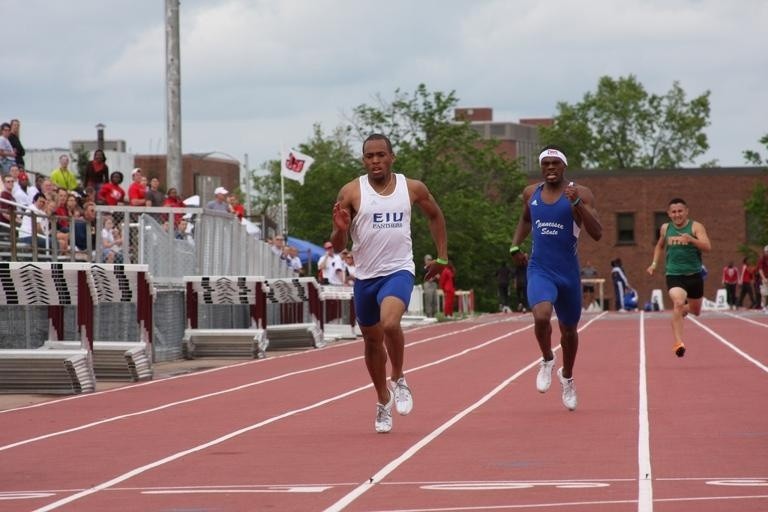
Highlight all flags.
[282,146,315,185]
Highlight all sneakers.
[374,387,395,434]
[536,349,556,393]
[672,340,686,357]
[556,365,578,411]
[388,377,414,417]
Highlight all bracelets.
[510,246,519,254]
[651,262,658,267]
[572,197,580,205]
[437,258,447,265]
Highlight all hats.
[214,186,229,195]
[130,167,142,176]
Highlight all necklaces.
[378,173,393,194]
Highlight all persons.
[494,262,514,311]
[330,134,448,433]
[580,260,600,310]
[207,186,229,212]
[227,194,244,221]
[438,259,455,318]
[510,143,604,411]
[513,257,528,313]
[424,255,437,317]
[317,242,354,287]
[611,258,629,312]
[266,235,303,276]
[647,197,712,356]
[722,247,767,310]
[0,119,188,264]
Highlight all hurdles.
[581,278,606,314]
[0,262,158,394]
[180,274,363,359]
[399,284,438,327]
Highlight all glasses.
[324,245,353,259]
[275,238,284,242]
[3,128,12,132]
[6,180,14,183]
